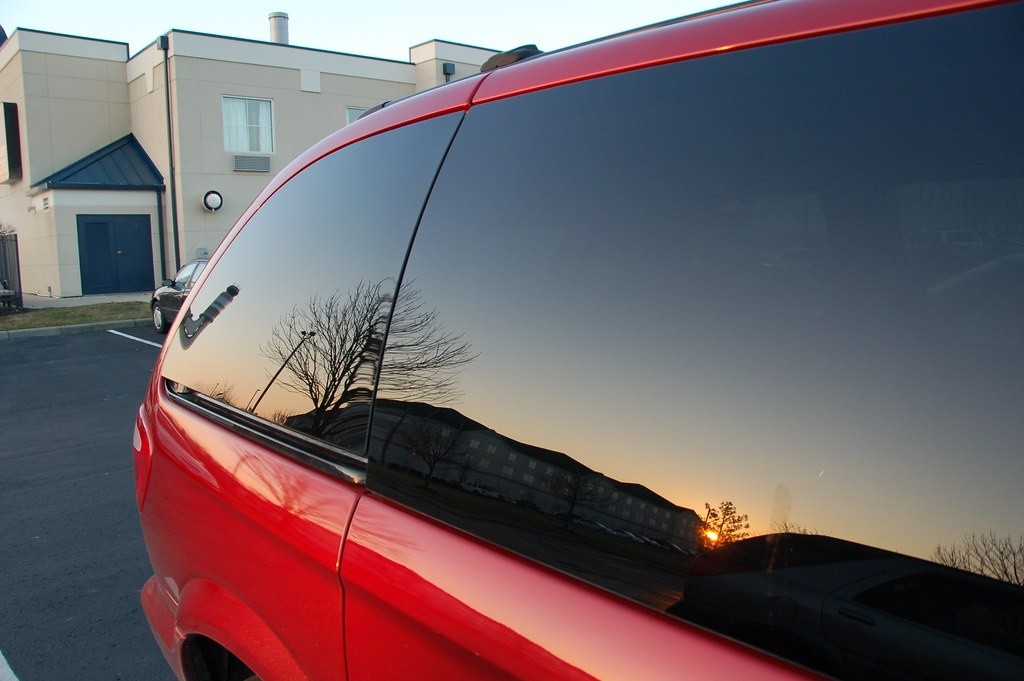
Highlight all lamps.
[201,188,224,213]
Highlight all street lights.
[244,389,260,412]
[209,383,220,397]
[249,330,319,416]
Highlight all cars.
[458,478,697,558]
[149,259,210,335]
[132,0,1024,681]
[664,532,1023,681]
